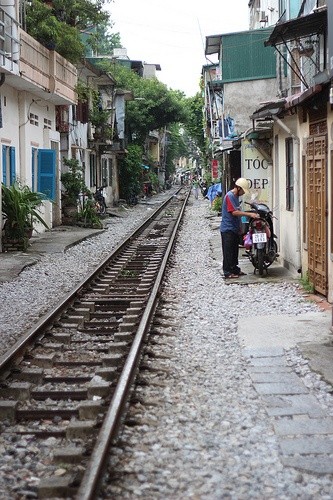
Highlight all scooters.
[92,185,108,215]
[201,182,208,196]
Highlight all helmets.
[235,178,250,193]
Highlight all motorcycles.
[241,201,280,278]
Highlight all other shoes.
[236,271,244,276]
[225,273,239,278]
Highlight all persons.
[193,179,198,199]
[219,177,256,277]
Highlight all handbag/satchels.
[243,232,252,250]
